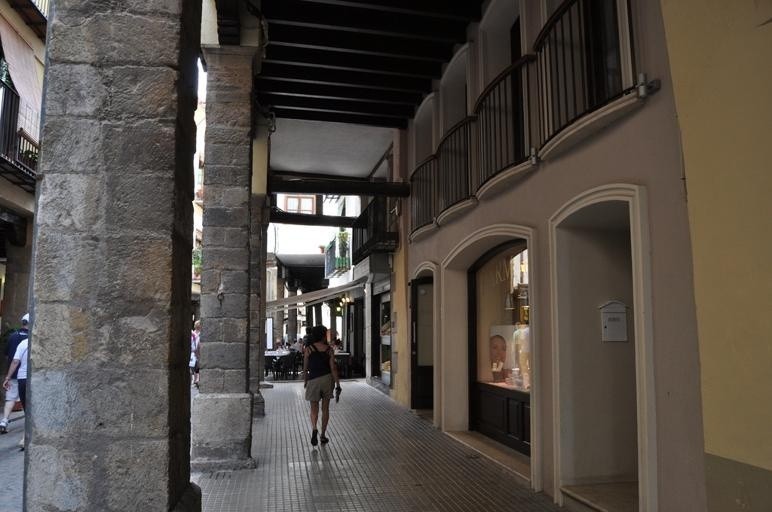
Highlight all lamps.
[336,296,355,316]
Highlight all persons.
[488,336,516,383]
[272,335,342,376]
[518,321,532,389]
[303,325,341,444]
[190,319,200,387]
[2,337,28,451]
[512,321,522,389]
[0,312,29,434]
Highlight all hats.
[21,314,30,322]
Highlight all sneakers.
[321,437,329,442]
[311,429,318,446]
[0,420,8,434]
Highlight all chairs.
[264,343,351,379]
